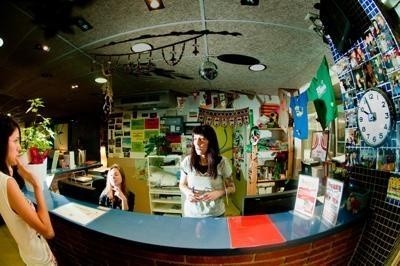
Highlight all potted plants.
[17,98,57,194]
[144,135,172,157]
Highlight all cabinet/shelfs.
[147,154,187,217]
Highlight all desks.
[57,160,109,206]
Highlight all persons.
[344,126,360,167]
[98,164,135,213]
[0,113,59,266]
[339,12,400,97]
[179,124,236,217]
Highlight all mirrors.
[253,127,294,184]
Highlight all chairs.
[125,191,136,212]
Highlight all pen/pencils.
[192,187,194,193]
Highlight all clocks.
[356,87,394,146]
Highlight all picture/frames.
[108,117,160,151]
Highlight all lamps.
[200,55,218,82]
